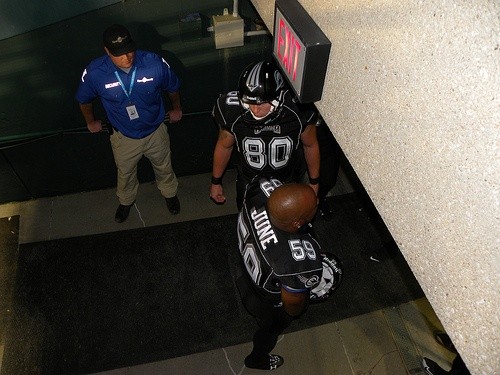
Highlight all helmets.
[237,60,285,105]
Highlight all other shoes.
[422,356,449,375]
[435,330,458,353]
[115,200,135,224]
[165,196,180,214]
[319,198,336,220]
[245,355,283,370]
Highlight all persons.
[235,174,323,370]
[76,24,182,223]
[211,61,320,212]
[423,333,471,375]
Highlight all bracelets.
[212,176,222,186]
[309,175,320,185]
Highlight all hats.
[104,24,136,57]
